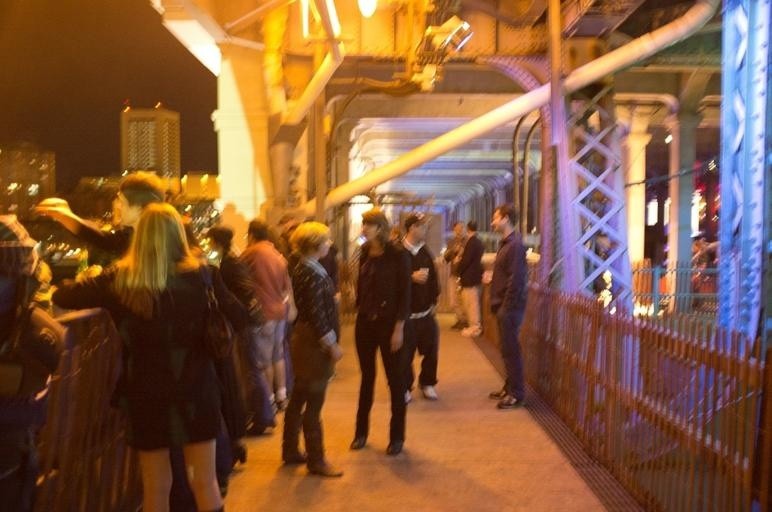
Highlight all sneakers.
[449,320,485,339]
[403,384,438,404]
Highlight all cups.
[420,267,429,273]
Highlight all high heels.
[234,442,248,469]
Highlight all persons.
[444,222,468,329]
[689,231,707,309]
[393,216,443,406]
[350,210,414,455]
[452,221,486,337]
[487,203,530,409]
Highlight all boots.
[350,417,371,449]
[305,418,344,478]
[282,413,308,463]
[384,421,408,454]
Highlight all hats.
[404,210,431,228]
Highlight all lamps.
[410,14,474,95]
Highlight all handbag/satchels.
[460,270,482,288]
[198,263,234,365]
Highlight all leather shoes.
[489,387,523,409]
[248,413,277,438]
